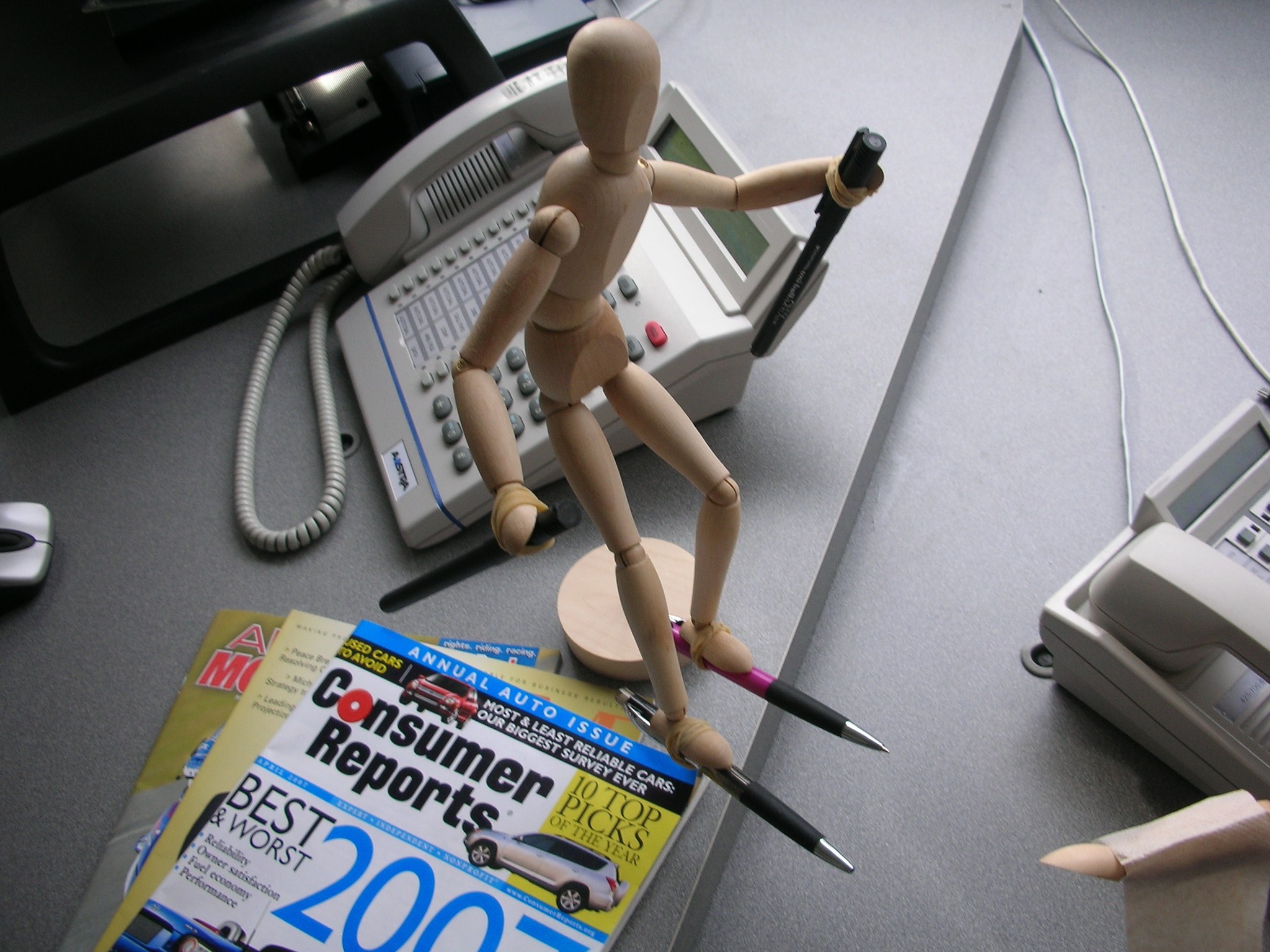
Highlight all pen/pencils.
[750,127,887,357]
[616,687,855,874]
[379,500,581,613]
[667,614,889,753]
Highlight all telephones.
[334,53,831,551]
[1037,385,1270,805]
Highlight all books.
[54,603,714,952]
